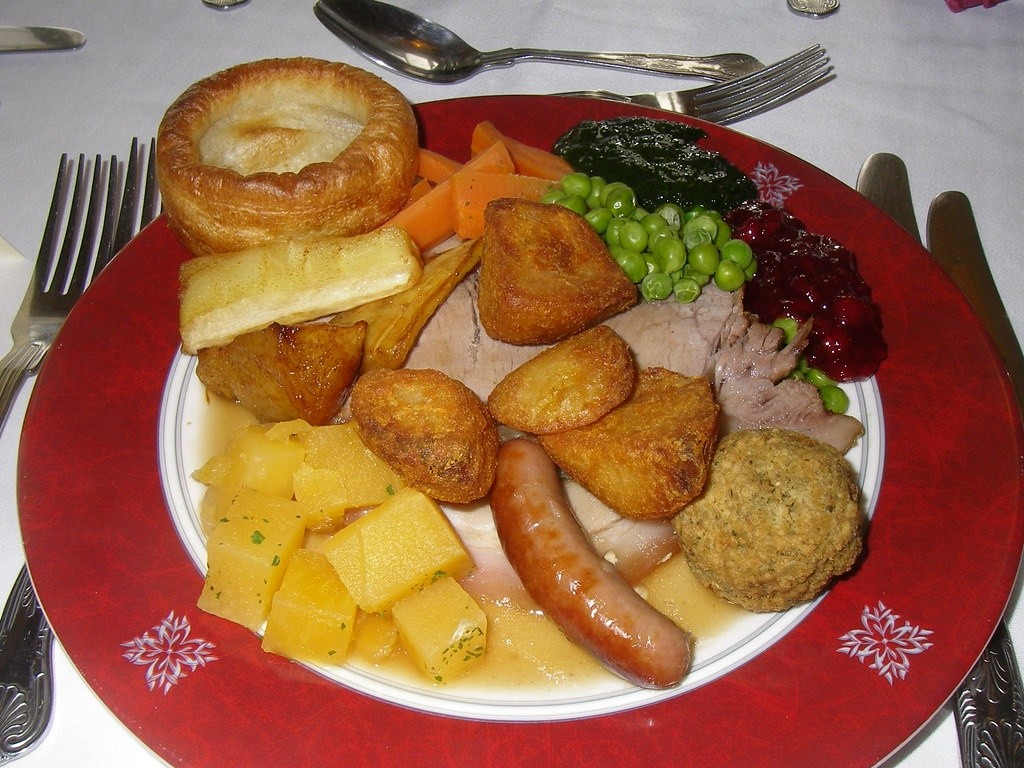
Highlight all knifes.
[856,154,1024,768]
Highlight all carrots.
[386,117,573,252]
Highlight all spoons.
[314,3,768,85]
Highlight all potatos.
[188,420,488,682]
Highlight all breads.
[155,54,418,257]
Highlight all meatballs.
[671,428,864,613]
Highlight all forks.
[1,137,156,751]
[3,152,116,425]
[553,42,832,127]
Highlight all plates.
[16,95,1024,767]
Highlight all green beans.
[541,171,850,414]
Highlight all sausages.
[486,437,692,690]
